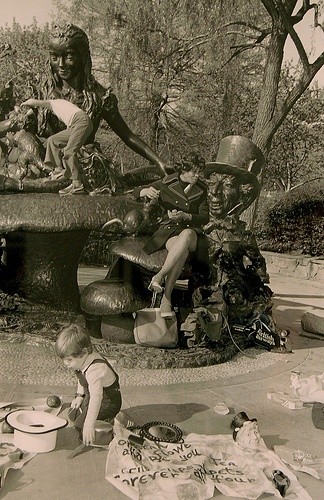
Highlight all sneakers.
[59,184,84,194]
[49,166,63,179]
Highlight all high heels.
[159,301,174,317]
[148,276,162,293]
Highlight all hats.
[5,409,68,453]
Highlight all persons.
[0,24,168,197]
[55,323,122,446]
[133,152,209,320]
[101,136,269,342]
[230,411,261,454]
[22,98,93,195]
